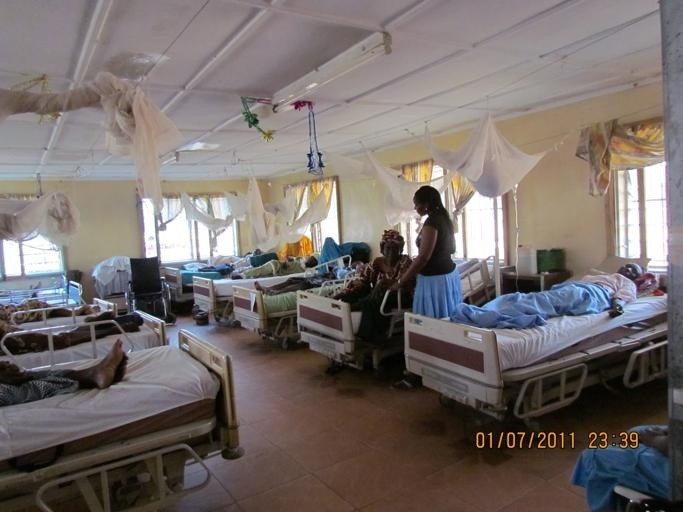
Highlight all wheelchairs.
[123,255,178,328]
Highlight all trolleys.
[1,276,171,374]
[395,295,667,437]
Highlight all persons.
[572,424,671,511]
[0,281,144,406]
[388,186,462,391]
[580,262,643,318]
[333,229,416,345]
[223,248,364,295]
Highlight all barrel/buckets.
[516,243,538,276]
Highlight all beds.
[1,272,82,311]
[193,260,328,321]
[0,311,165,370]
[405,256,670,433]
[160,258,247,301]
[295,258,505,378]
[0,297,115,333]
[229,273,361,350]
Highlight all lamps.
[271,32,393,113]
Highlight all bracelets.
[398,278,402,290]
